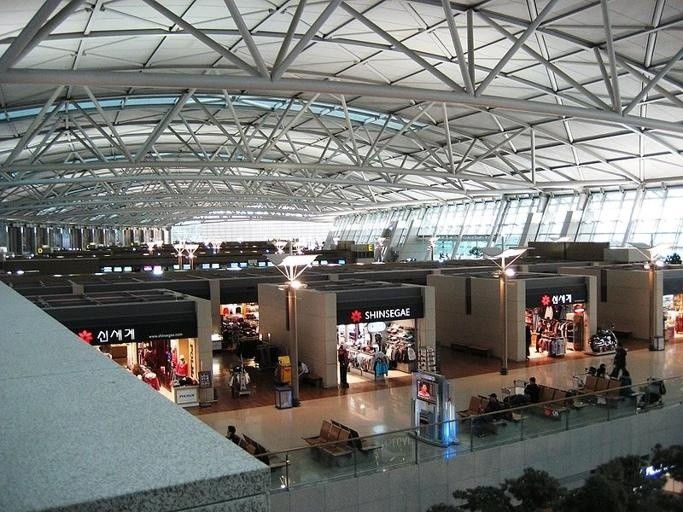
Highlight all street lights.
[262,253,319,407]
[292,242,304,255]
[628,240,675,351]
[145,239,222,271]
[273,240,286,254]
[428,234,440,260]
[376,236,386,263]
[332,236,340,248]
[481,236,528,372]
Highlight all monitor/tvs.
[417,379,438,406]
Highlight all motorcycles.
[588,318,620,353]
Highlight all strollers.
[635,377,666,412]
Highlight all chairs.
[302,419,382,466]
[234,434,280,465]
[456,376,637,434]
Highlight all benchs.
[308,375,322,387]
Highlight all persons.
[582,364,636,402]
[514,377,539,414]
[525,321,532,356]
[175,355,188,380]
[609,343,629,379]
[224,426,240,446]
[297,360,309,385]
[471,393,501,438]
[530,320,544,353]
[338,348,349,384]
[417,383,431,400]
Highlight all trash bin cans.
[650,335,664,351]
[275,385,293,409]
